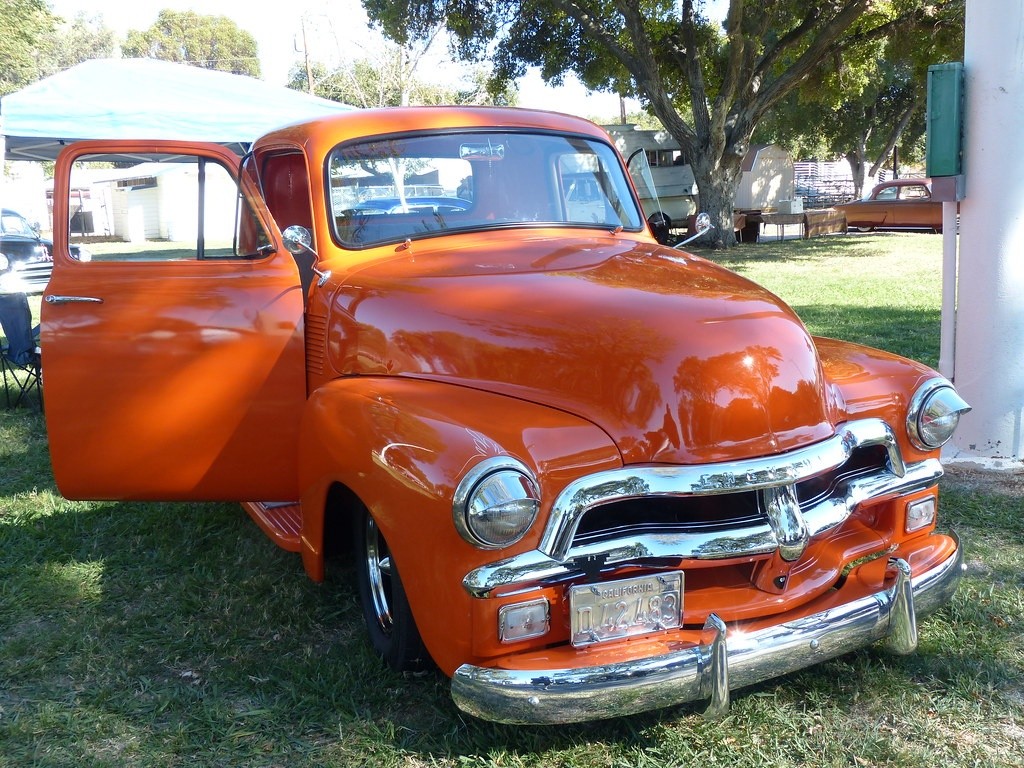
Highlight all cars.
[39,105,973,729]
[0,208,92,297]
[829,178,960,236]
[353,196,473,217]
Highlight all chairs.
[0,292,44,415]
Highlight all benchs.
[337,217,522,247]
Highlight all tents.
[1,56,364,259]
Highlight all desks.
[757,209,848,243]
